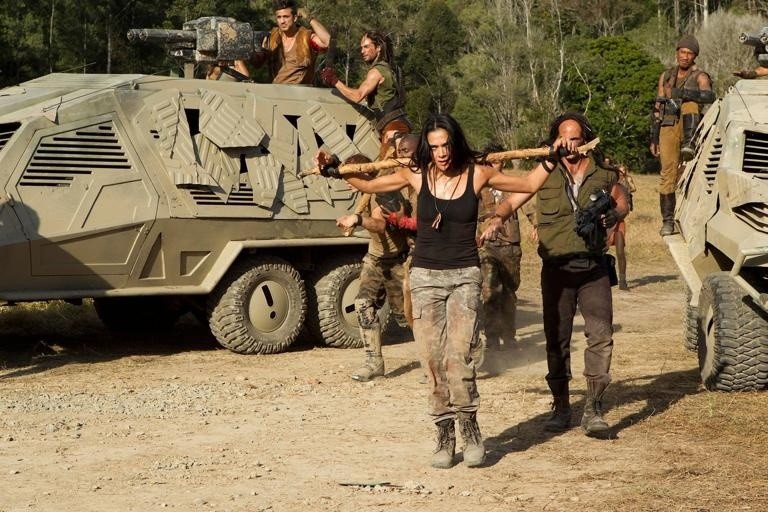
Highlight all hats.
[676,33,699,55]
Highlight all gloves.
[320,68,338,86]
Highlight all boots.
[581,379,609,433]
[458,418,486,466]
[545,373,571,432]
[681,113,700,158]
[660,191,676,235]
[620,274,629,290]
[431,419,456,468]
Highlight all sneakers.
[353,355,384,381]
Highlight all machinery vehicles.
[657,27,768,392]
[0,16,432,353]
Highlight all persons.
[649,34,714,236]
[316,118,419,381]
[731,66,766,79]
[478,147,540,352]
[619,166,636,193]
[259,4,330,85]
[605,156,613,165]
[477,106,629,438]
[313,114,578,470]
[608,220,630,291]
[317,30,413,139]
[205,59,251,83]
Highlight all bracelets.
[542,160,553,174]
[491,214,505,225]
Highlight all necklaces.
[431,163,462,230]
